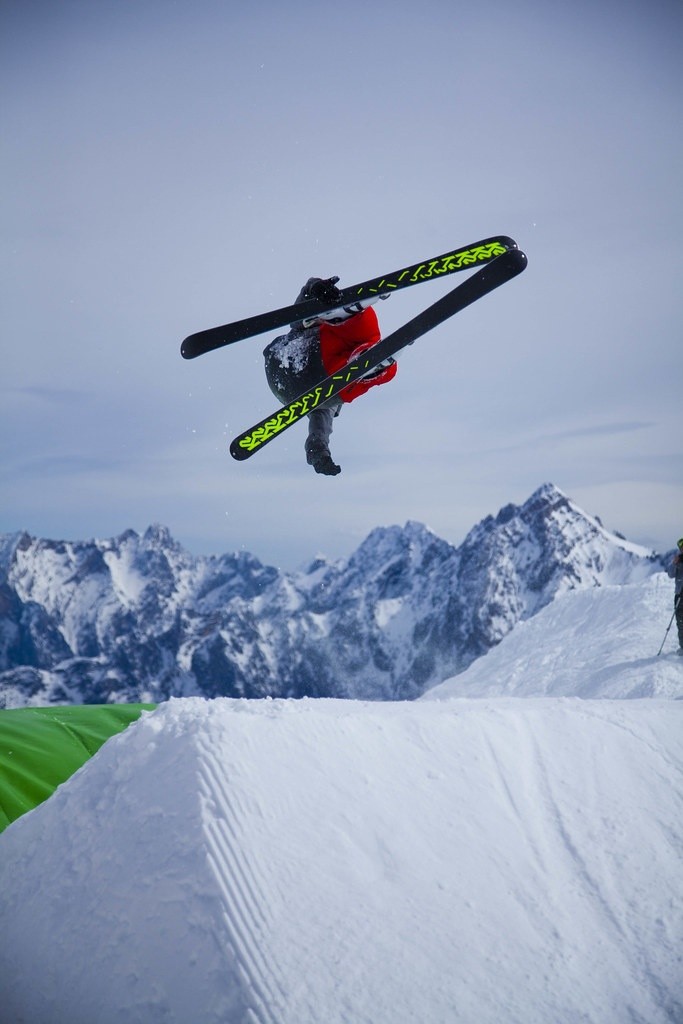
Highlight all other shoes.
[676,648,683,655]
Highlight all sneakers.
[318,294,379,325]
[350,339,408,380]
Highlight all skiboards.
[178,234,527,462]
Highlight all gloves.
[310,275,340,297]
[315,456,341,477]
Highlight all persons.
[668,539,683,656]
[263,276,397,477]
